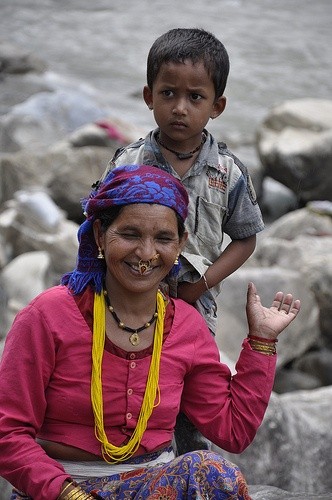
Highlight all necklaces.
[157,133,206,161]
[101,284,160,346]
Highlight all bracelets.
[59,480,93,500]
[202,274,211,293]
[247,332,278,355]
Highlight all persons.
[0,164,302,500]
[103,26,264,456]
